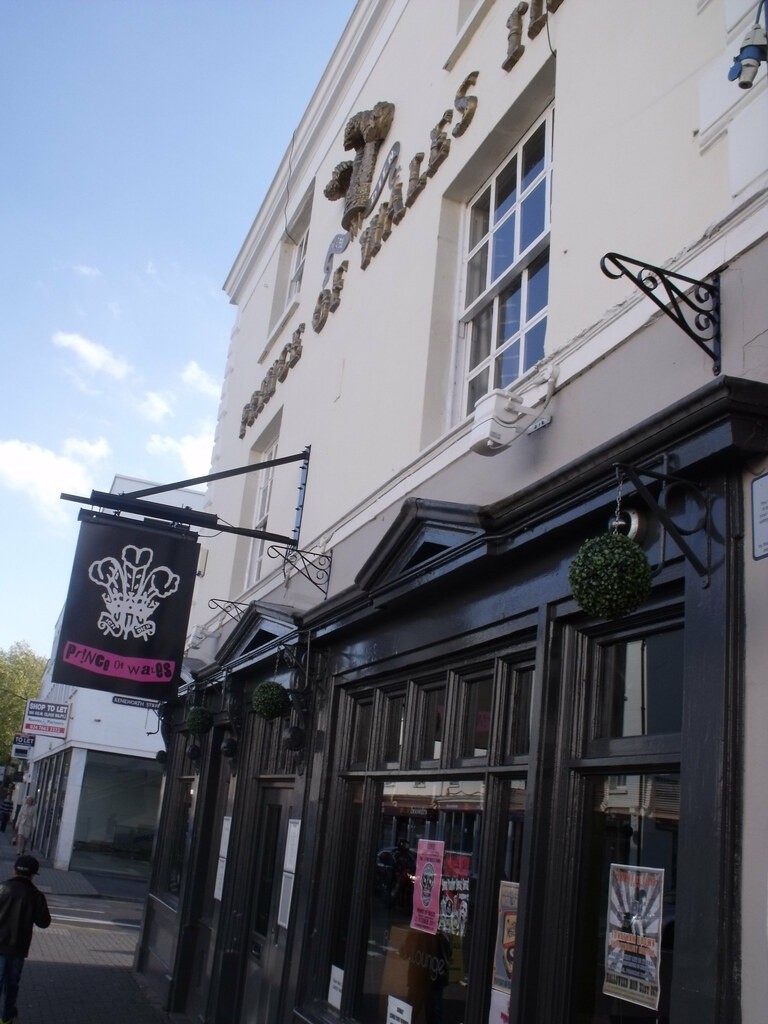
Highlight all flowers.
[251,681,289,720]
[568,532,653,619]
[186,707,214,737]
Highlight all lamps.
[470,363,558,458]
[188,624,221,650]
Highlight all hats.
[14,855,40,877]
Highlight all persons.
[0,793,13,833]
[15,796,37,855]
[619,890,647,973]
[398,930,454,1024]
[0,855,52,1024]
